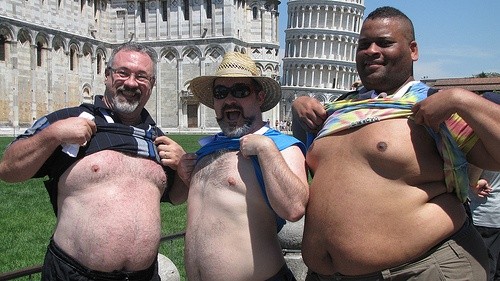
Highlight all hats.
[190,52,282,112]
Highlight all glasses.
[109,67,152,82]
[213,83,256,100]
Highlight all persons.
[291,6,500,281]
[177,52,309,281]
[0,41,188,281]
[267,118,293,135]
[468,93,500,281]
